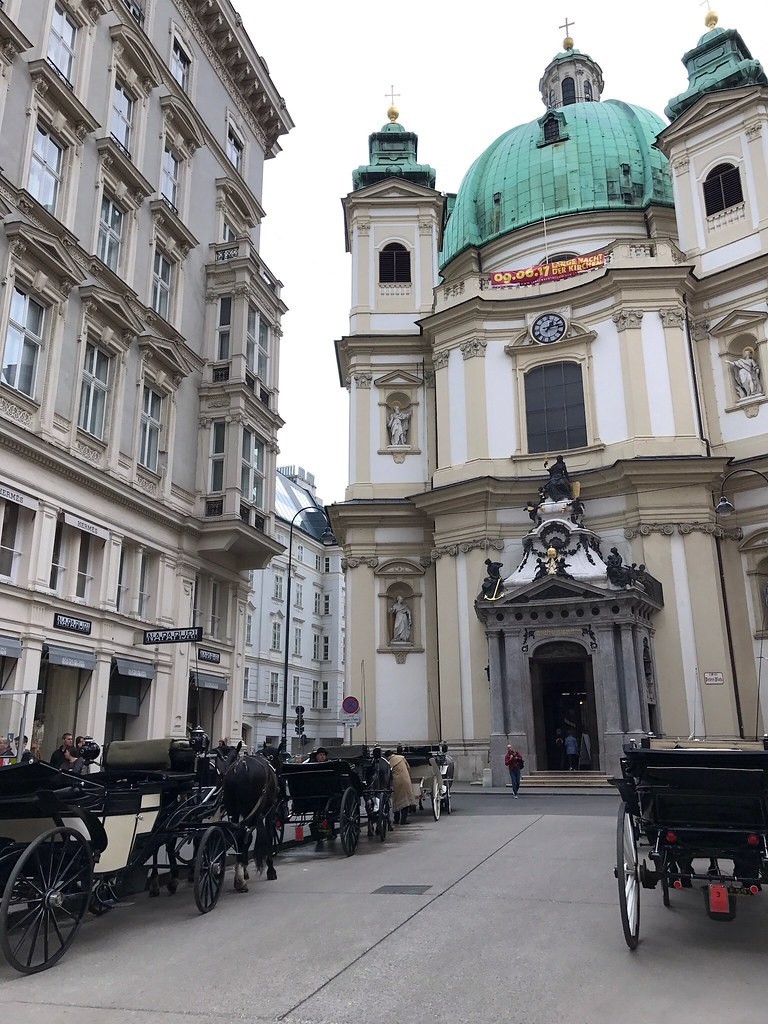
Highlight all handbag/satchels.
[514,752,525,769]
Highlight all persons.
[607,547,645,587]
[370,748,393,786]
[532,558,551,583]
[555,729,579,771]
[0,736,16,765]
[505,745,522,799]
[538,486,549,505]
[295,754,303,763]
[31,713,48,758]
[724,351,759,397]
[523,501,545,534]
[14,734,34,763]
[544,455,574,502]
[567,497,586,529]
[315,747,329,761]
[50,733,90,775]
[384,750,417,825]
[482,558,503,598]
[554,558,575,580]
[219,739,226,747]
[389,595,411,642]
[387,406,412,445]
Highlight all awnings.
[0,638,22,659]
[194,673,228,691]
[116,659,156,680]
[46,645,96,670]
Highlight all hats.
[384,750,393,758]
[315,748,328,756]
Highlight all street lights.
[281,507,338,762]
[714,468,768,516]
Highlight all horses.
[416,745,455,813]
[147,739,289,898]
[364,751,395,843]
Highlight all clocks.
[532,314,565,343]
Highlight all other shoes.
[393,821,399,824]
[512,791,517,799]
[401,822,409,825]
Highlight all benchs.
[608,738,768,859]
[0,738,447,876]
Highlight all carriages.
[0,738,454,974]
[607,738,768,950]
